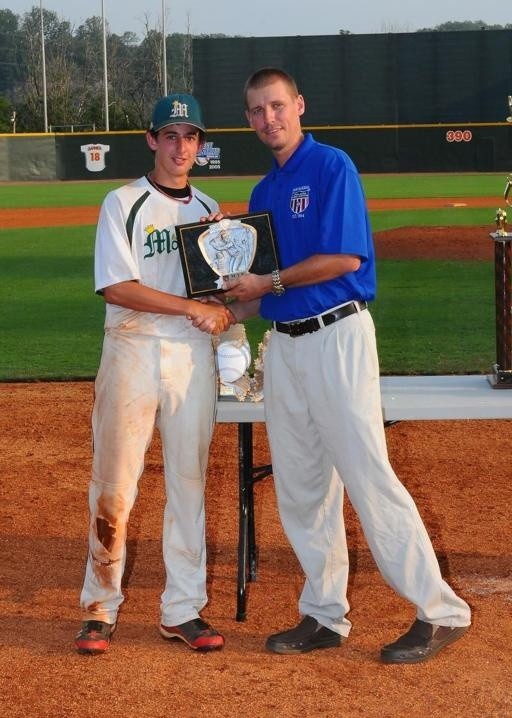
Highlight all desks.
[218,383,512,623]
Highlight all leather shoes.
[267,615,346,656]
[381,618,470,663]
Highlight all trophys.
[503,173,512,208]
[494,207,508,238]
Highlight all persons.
[208,234,243,272]
[180,65,472,667]
[72,91,234,653]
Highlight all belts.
[273,300,367,338]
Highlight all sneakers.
[73,619,116,656]
[159,618,225,651]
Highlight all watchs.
[272,268,285,298]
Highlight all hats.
[149,93,207,134]
